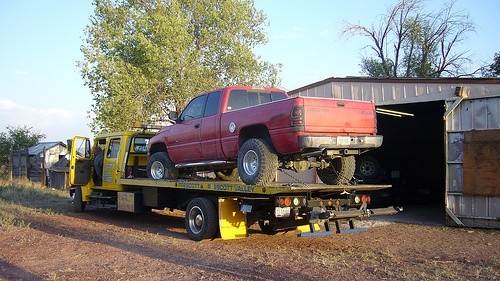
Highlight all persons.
[91,147,104,179]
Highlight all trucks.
[68,124,405,243]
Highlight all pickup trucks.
[146,84,384,185]
